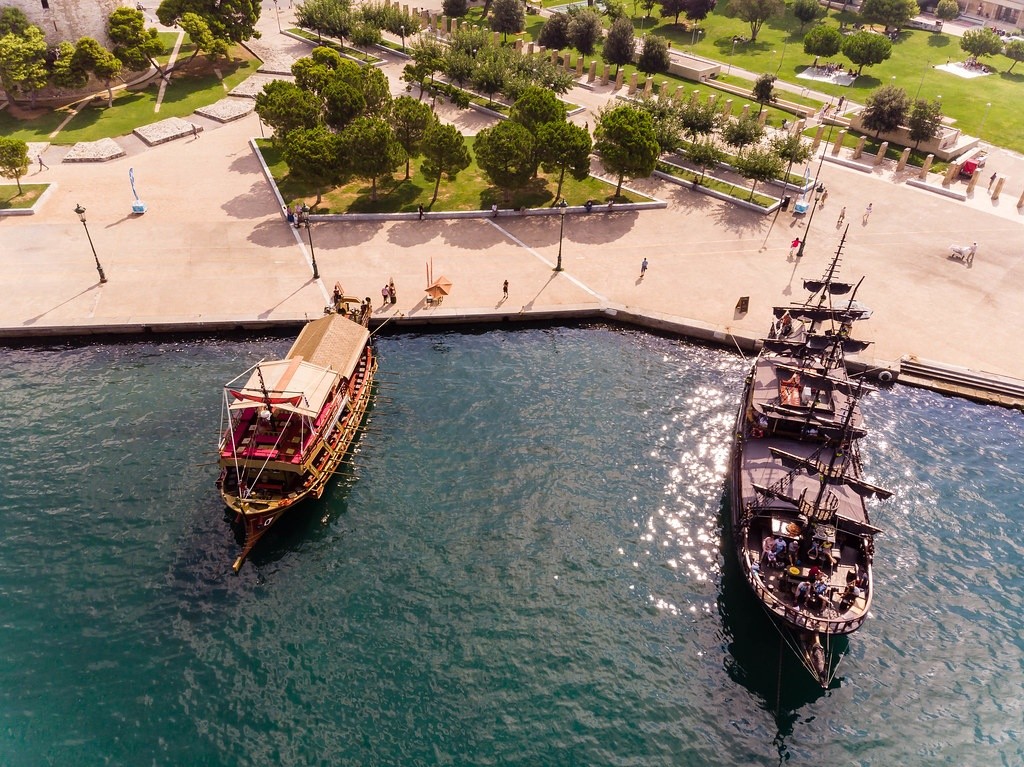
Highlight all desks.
[280,485,283,488]
[256,483,286,495]
[221,401,334,465]
[777,564,811,585]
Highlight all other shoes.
[503,296,505,298]
[506,297,508,299]
[386,301,387,303]
[384,303,385,305]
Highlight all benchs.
[258,445,277,450]
[762,528,771,560]
[250,492,273,499]
[261,432,282,436]
[269,421,291,427]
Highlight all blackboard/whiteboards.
[740,297,749,312]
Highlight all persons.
[239,481,255,506]
[790,238,803,255]
[360,297,371,317]
[640,258,648,277]
[427,293,433,303]
[333,285,340,305]
[820,190,828,207]
[988,172,997,189]
[945,55,951,66]
[338,307,347,317]
[768,313,792,339]
[691,175,699,191]
[852,23,865,30]
[731,34,749,42]
[586,201,592,213]
[491,202,498,217]
[962,56,990,72]
[382,283,396,305]
[837,97,845,111]
[810,57,859,78]
[37,154,49,172]
[966,243,977,264]
[286,203,302,228]
[887,27,900,43]
[419,203,425,220]
[668,40,672,50]
[863,203,872,223]
[749,532,869,616]
[819,101,831,119]
[191,123,201,140]
[607,198,614,212]
[503,279,509,299]
[838,206,846,224]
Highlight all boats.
[218,314,399,571]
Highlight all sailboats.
[730,224,894,690]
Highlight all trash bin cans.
[784,195,791,208]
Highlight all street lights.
[916,61,935,99]
[401,25,406,54]
[74,203,108,283]
[556,198,568,271]
[641,16,645,31]
[692,24,696,44]
[299,203,320,279]
[697,31,702,43]
[796,183,823,256]
[728,40,737,74]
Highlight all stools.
[791,585,798,594]
[307,417,315,422]
[299,428,308,436]
[242,438,251,445]
[291,437,301,448]
[286,448,295,458]
[249,425,257,433]
[236,446,245,453]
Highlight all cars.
[1001,36,1024,44]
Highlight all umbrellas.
[424,275,452,297]
